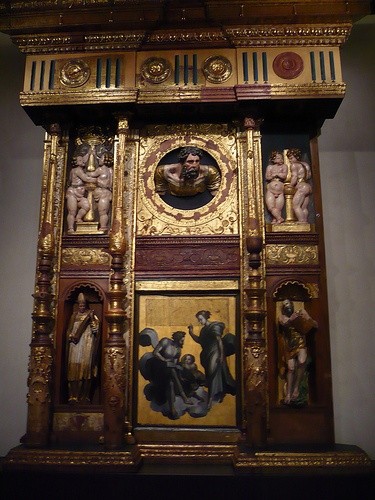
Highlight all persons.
[58,280,105,431]
[273,296,318,407]
[58,139,316,244]
